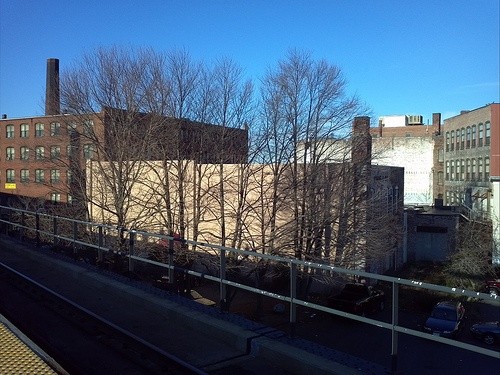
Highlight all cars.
[471,317,500,346]
[485,278,500,300]
[327,283,385,319]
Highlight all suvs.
[425,299,466,338]
